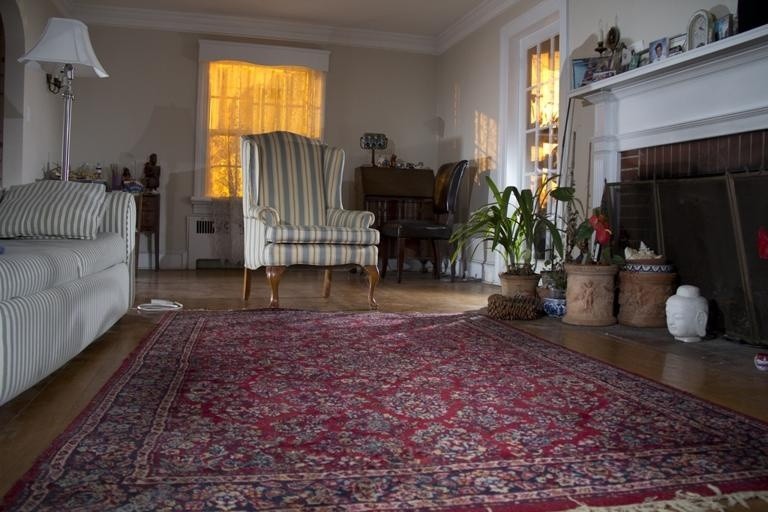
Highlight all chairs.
[239,130,380,311]
[380,159,469,284]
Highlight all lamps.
[46,72,62,94]
[359,132,388,167]
[17,16,110,180]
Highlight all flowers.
[550,187,613,264]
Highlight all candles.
[597,28,603,41]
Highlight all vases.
[617,259,676,328]
[543,298,567,320]
[561,262,618,327]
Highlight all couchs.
[0,191,136,407]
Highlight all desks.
[135,193,160,277]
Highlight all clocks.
[686,9,714,51]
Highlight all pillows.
[0,179,109,240]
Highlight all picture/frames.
[638,50,650,67]
[648,36,669,64]
[669,32,688,58]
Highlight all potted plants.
[540,254,567,288]
[447,174,564,299]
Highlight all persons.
[653,42,667,64]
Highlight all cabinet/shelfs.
[352,166,438,278]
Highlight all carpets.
[0,307,768,512]
